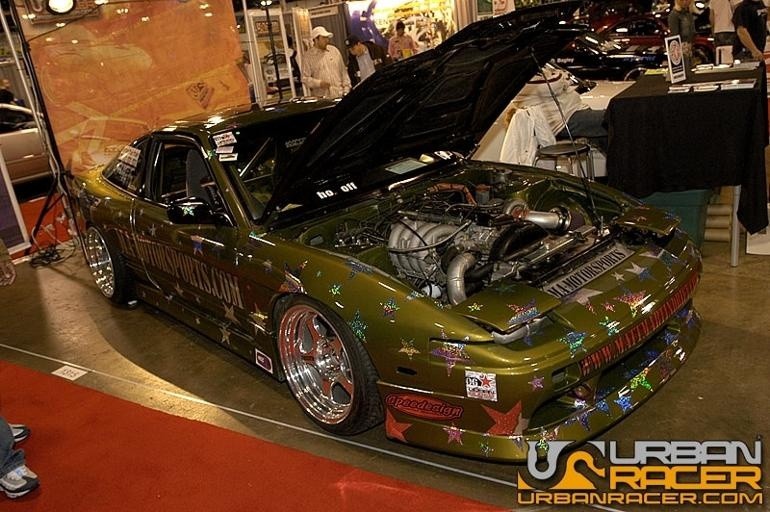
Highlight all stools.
[532,140,594,182]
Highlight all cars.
[0,102,58,185]
[550,10,718,80]
[63,0,704,465]
[258,47,301,88]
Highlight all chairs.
[185,148,210,207]
[270,122,311,191]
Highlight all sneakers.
[8,423,29,442]
[0,464,41,500]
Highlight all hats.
[344,34,361,48]
[312,26,334,41]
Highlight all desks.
[609,60,765,270]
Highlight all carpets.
[0,358,516,510]
[6,194,75,262]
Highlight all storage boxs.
[642,189,713,253]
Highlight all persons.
[388,21,421,62]
[511,63,607,161]
[667,0,768,62]
[300,26,352,100]
[0,416,40,499]
[343,34,393,89]
